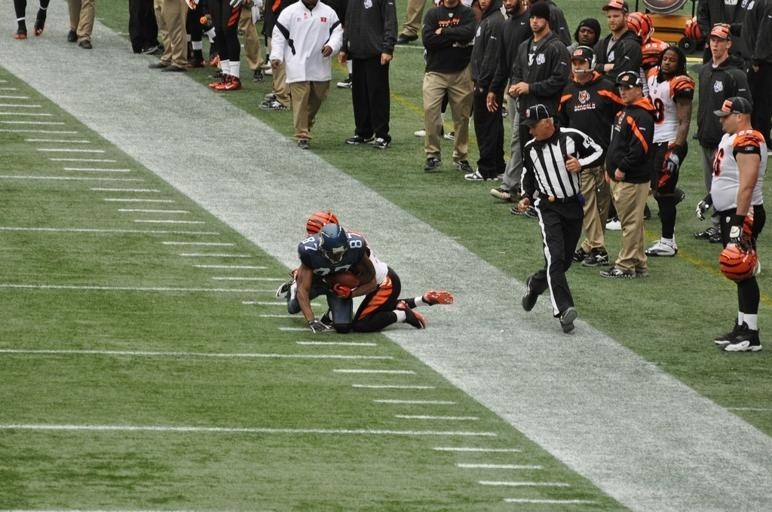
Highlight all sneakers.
[693,224,722,244]
[644,234,679,258]
[412,121,687,229]
[130,31,420,151]
[598,264,649,281]
[66,28,94,50]
[31,9,48,36]
[421,290,456,306]
[320,307,334,324]
[712,314,764,353]
[394,299,428,330]
[520,274,538,313]
[557,306,580,334]
[14,25,29,41]
[573,245,609,266]
[275,280,292,299]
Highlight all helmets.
[305,211,341,236]
[716,240,756,283]
[626,11,655,45]
[320,223,348,253]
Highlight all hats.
[569,47,594,63]
[601,0,629,14]
[577,17,600,41]
[708,22,733,41]
[613,70,644,87]
[711,95,754,120]
[528,3,552,22]
[518,103,552,127]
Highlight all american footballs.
[331,273,360,288]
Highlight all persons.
[399,1,772,279]
[275,226,377,334]
[519,105,604,334]
[127,2,400,148]
[696,98,770,352]
[13,0,51,40]
[65,0,96,49]
[290,210,452,332]
